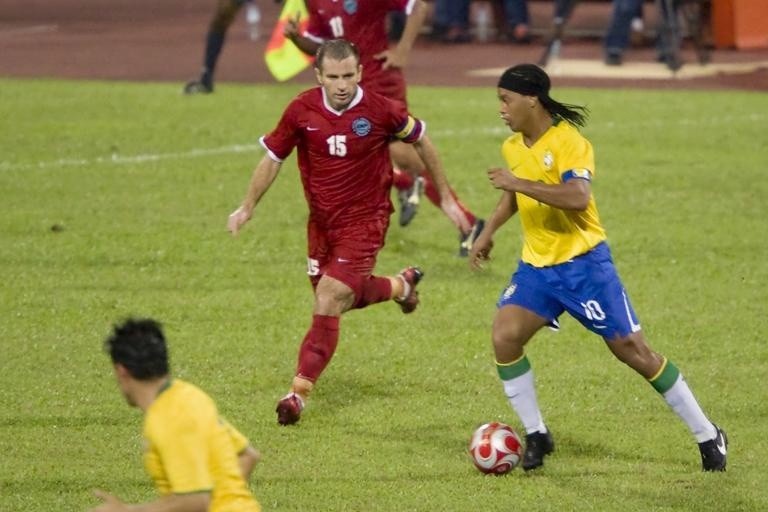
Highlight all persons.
[469,63,728,469]
[227,37,471,422]
[89,318,260,512]
[432,0,530,41]
[283,0,485,258]
[185,0,244,93]
[605,1,677,67]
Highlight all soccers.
[468,421,525,478]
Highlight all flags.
[265,0,317,82]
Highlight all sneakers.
[460,218,486,256]
[698,423,728,472]
[393,267,424,314]
[276,395,302,426]
[522,424,555,472]
[397,176,424,226]
